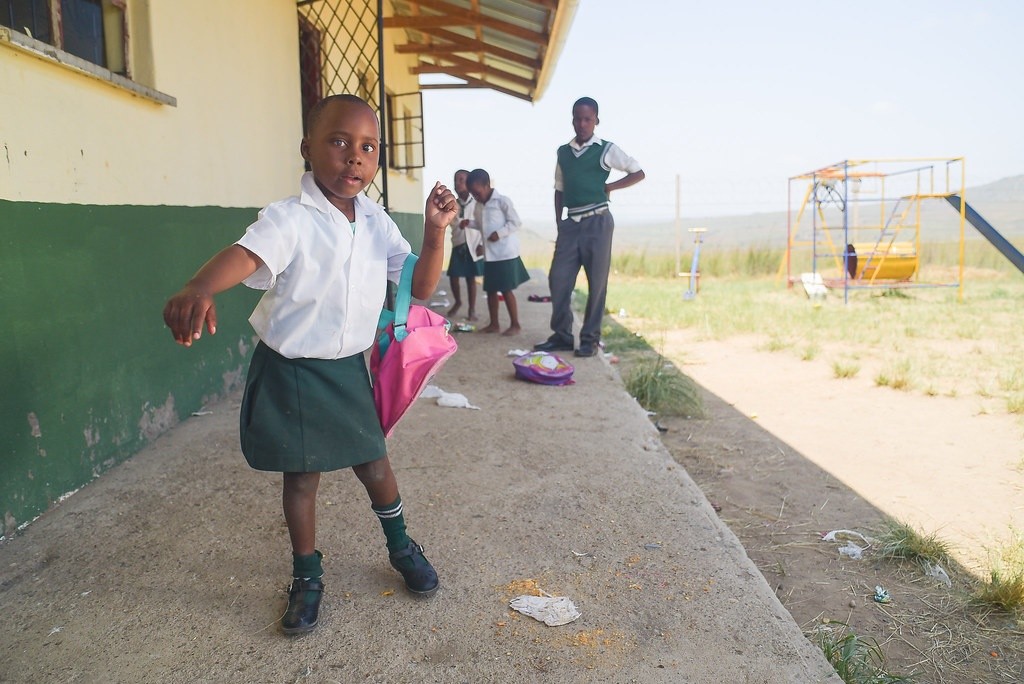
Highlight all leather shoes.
[575,342,597,356]
[535,341,574,350]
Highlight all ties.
[454,198,473,218]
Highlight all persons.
[458,168,530,338]
[445,169,482,323]
[533,95,646,359]
[160,94,461,637]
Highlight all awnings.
[390,0,579,106]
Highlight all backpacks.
[513,351,575,386]
[369,253,457,439]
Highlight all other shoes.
[281,580,325,633]
[390,539,439,596]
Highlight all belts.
[571,206,609,219]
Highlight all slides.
[946,196,1024,273]
